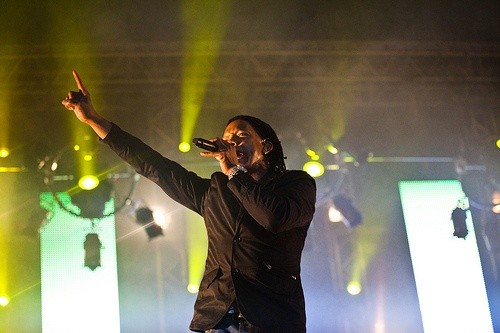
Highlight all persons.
[61,68,316,333]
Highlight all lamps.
[332,194,364,228]
[83,234,105,270]
[135,208,165,241]
[452,208,470,239]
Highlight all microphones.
[194,137,218,153]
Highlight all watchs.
[227,165,248,181]
